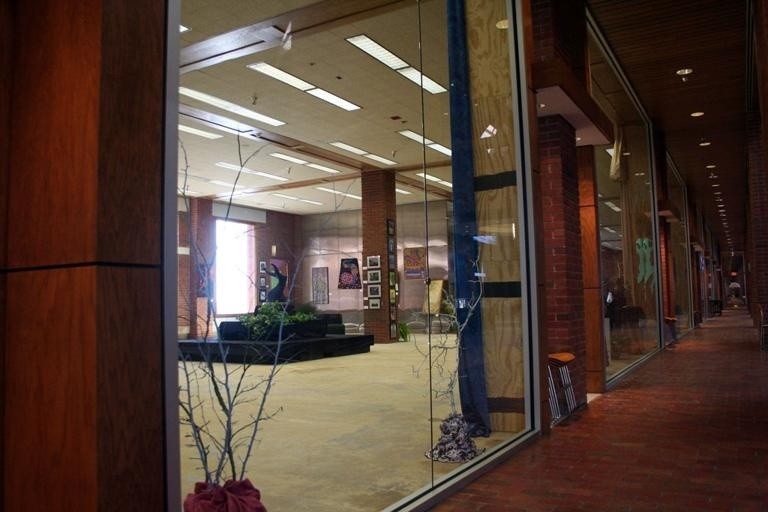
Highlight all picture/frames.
[388,219,397,339]
[367,256,381,309]
[258,259,266,302]
